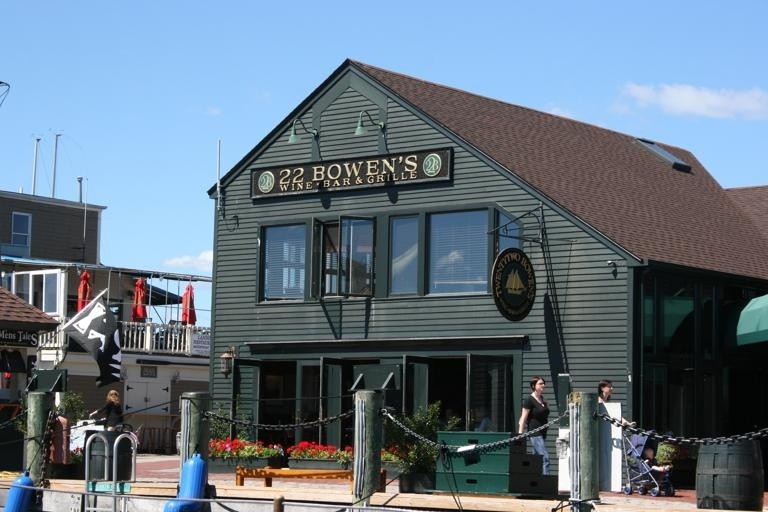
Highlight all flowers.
[208,437,284,460]
[344,447,402,463]
[287,441,344,464]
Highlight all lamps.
[288,119,318,145]
[354,110,385,137]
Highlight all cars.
[67,423,105,455]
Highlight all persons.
[89,390,124,432]
[518,377,550,476]
[596,380,637,428]
[473,406,496,432]
[643,448,675,487]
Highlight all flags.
[61,289,121,389]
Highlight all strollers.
[618,419,675,498]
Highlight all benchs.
[235,466,386,495]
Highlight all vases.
[207,460,268,473]
[348,462,405,479]
[288,457,348,470]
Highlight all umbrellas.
[78,269,92,312]
[132,277,147,322]
[182,283,196,325]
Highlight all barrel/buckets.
[695,442,764,511]
[85,429,132,481]
